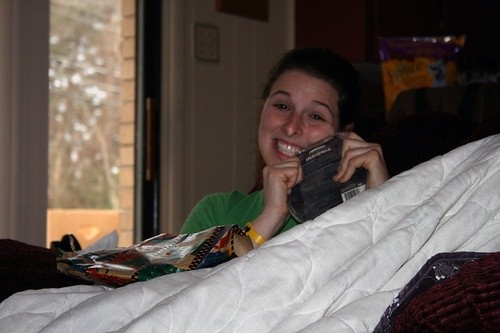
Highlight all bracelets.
[240,221,266,246]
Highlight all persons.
[179,45,391,254]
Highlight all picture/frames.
[215,0,269,22]
